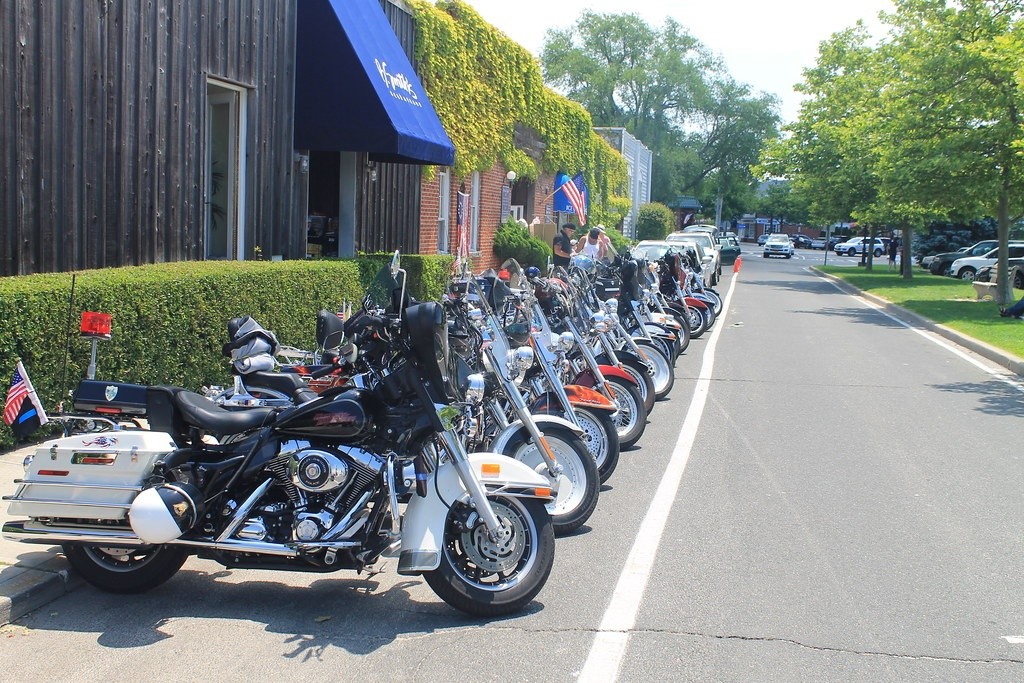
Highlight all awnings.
[294,1,456,167]
[553,173,589,216]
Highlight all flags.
[560,175,590,225]
[456,192,470,266]
[4,362,34,425]
[451,259,462,271]
[683,214,693,224]
[345,305,350,320]
[336,302,344,321]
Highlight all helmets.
[525,266,540,282]
[128,481,203,544]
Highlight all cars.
[919,240,1024,292]
[630,239,713,290]
[756,233,852,255]
[679,223,742,273]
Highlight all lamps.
[294,153,309,173]
[366,163,377,181]
[507,170,516,189]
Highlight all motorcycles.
[0,251,561,620]
[197,244,724,538]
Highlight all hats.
[595,224,605,233]
[562,222,576,230]
[589,229,600,239]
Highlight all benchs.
[973,265,1020,302]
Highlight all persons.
[888,237,902,272]
[575,228,603,259]
[998,296,1024,317]
[552,222,576,276]
[593,224,611,260]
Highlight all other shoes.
[998,306,1010,317]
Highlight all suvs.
[665,230,724,286]
[833,236,885,258]
[762,233,795,259]
[880,237,893,255]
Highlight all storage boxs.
[7,431,178,520]
[446,278,494,304]
